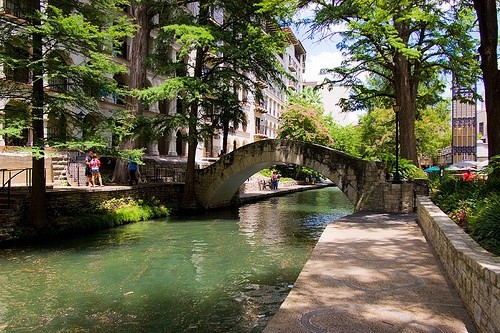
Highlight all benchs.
[262,181,272,190]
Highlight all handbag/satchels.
[92,166,99,172]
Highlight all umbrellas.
[424,166,440,171]
[445,160,489,173]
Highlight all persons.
[85,149,102,187]
[271,171,282,190]
[304,171,316,184]
[128,155,138,185]
[464,169,475,181]
[218,150,226,159]
